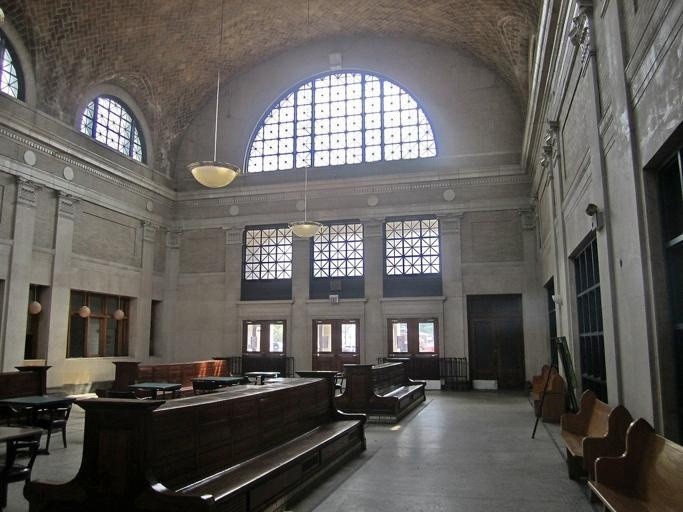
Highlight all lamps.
[586,204,597,215]
[287,0,323,239]
[78,292,91,318]
[186,1,242,188]
[113,296,124,320]
[28,286,41,314]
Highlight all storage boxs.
[473,379,498,391]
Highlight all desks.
[246,372,280,384]
[0,425,42,509]
[128,380,182,400]
[7,393,78,456]
[190,376,244,394]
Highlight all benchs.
[585,418,683,512]
[560,389,634,479]
[19,371,370,512]
[529,365,566,421]
[334,356,425,420]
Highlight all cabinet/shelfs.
[0,362,52,399]
[112,358,230,388]
